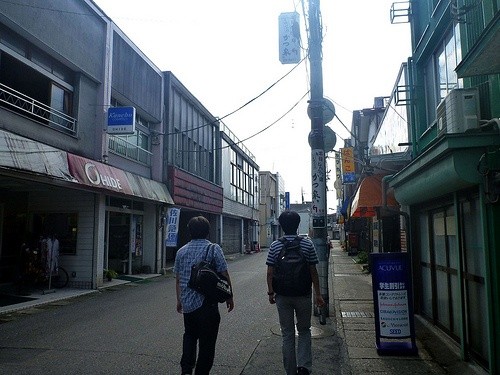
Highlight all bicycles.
[20,260,69,292]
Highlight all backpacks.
[273,236,312,297]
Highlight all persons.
[266,211,325,375]
[173,216,234,375]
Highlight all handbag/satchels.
[187,243,231,303]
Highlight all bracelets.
[268,292,274,295]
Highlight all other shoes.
[297,367,311,375]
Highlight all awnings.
[351,175,399,217]
